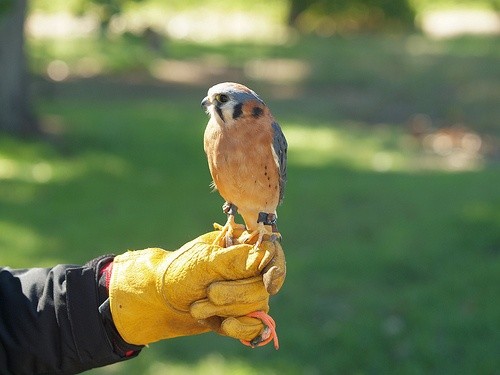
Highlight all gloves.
[109,228,287,344]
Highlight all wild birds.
[200,81,288,253]
